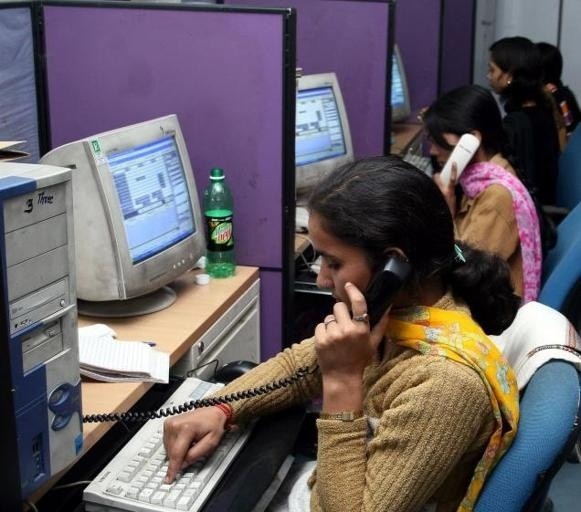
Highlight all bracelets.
[216,403,230,428]
[318,410,363,421]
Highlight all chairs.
[455,353,581,512]
[535,199,581,317]
[541,118,580,221]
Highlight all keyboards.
[402,130,437,178]
[82,376,260,512]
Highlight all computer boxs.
[0,161,83,500]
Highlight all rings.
[326,318,335,326]
[354,314,371,323]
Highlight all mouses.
[215,360,259,381]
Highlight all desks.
[11,255,267,510]
[385,116,428,163]
[290,190,314,263]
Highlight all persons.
[535,42,580,157]
[417,83,543,305]
[164,152,520,512]
[486,36,556,202]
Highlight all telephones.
[349,258,412,331]
[440,133,481,188]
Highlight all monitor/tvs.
[387,44,412,144]
[34,114,206,318]
[295,72,355,207]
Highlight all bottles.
[202,167,238,280]
